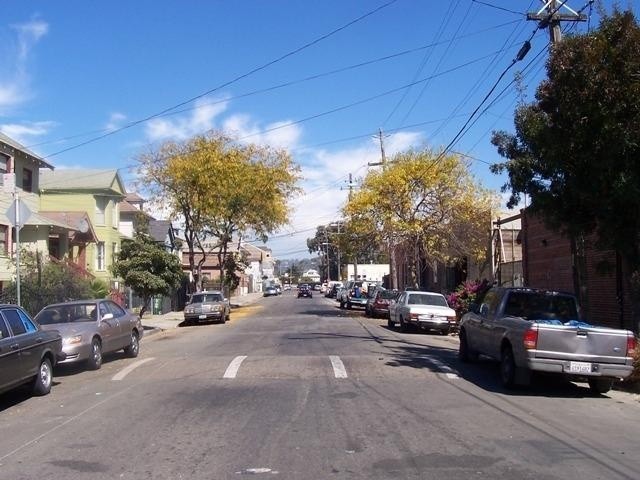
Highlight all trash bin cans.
[153,293,163,315]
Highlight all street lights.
[321,223,360,280]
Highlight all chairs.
[214,297,219,302]
[91,309,102,319]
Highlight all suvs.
[337,281,382,308]
[314,281,345,297]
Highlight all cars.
[366,289,404,316]
[264,283,291,297]
[0,302,67,397]
[34,298,144,370]
[296,283,312,298]
[184,289,231,326]
[389,291,457,335]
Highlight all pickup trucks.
[459,286,636,396]
